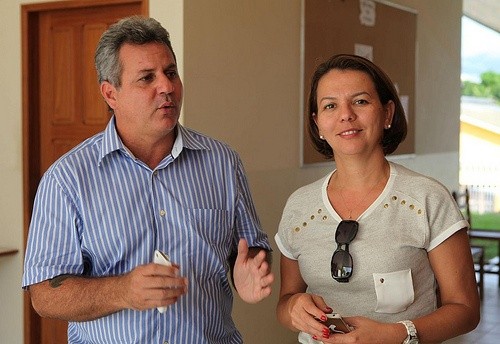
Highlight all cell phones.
[153,250,172,313]
[313,313,351,334]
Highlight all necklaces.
[334,185,379,218]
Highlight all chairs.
[452,189,500,295]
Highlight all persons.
[22,15,275,344]
[273,51,481,344]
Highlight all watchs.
[396,320,419,344]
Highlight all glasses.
[331,220,359,283]
